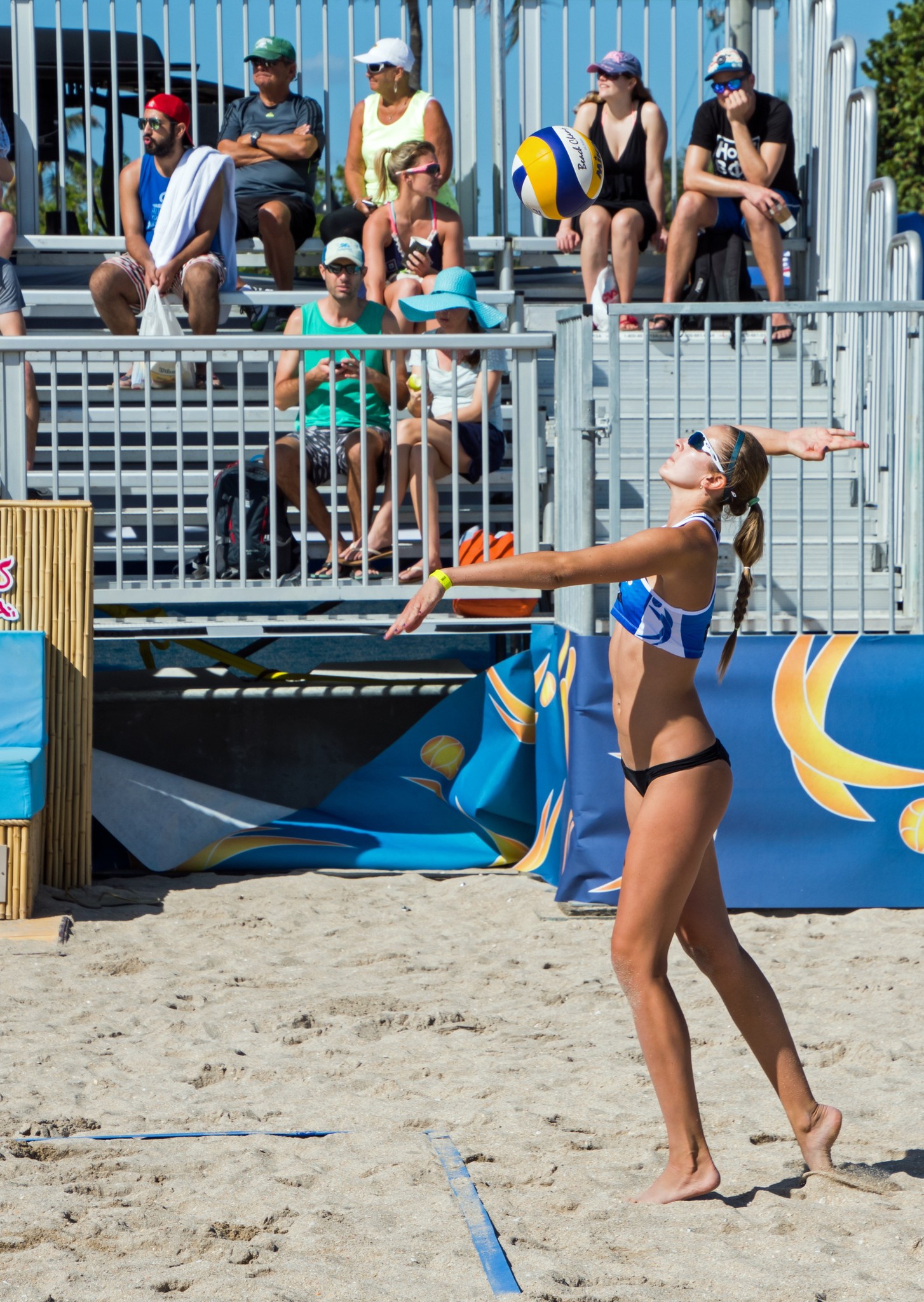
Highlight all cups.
[401,234,432,271]
[769,203,797,231]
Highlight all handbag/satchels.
[131,284,196,390]
[453,518,539,617]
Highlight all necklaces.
[386,102,402,120]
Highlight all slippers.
[337,542,393,565]
[309,557,345,578]
[762,322,795,344]
[619,316,639,330]
[350,566,383,580]
[398,566,433,584]
[642,317,671,330]
[197,373,224,389]
[107,373,133,390]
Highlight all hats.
[398,266,507,329]
[586,50,642,79]
[145,94,194,147]
[704,46,749,81]
[244,37,296,64]
[353,37,416,73]
[322,236,366,267]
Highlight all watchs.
[250,130,262,148]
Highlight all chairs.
[0,627,51,921]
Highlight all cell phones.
[361,199,377,208]
[401,241,427,269]
[335,362,348,369]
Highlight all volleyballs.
[511,124,605,220]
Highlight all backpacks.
[174,451,310,587]
[670,229,764,331]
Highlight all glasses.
[138,117,177,130]
[597,71,622,80]
[711,76,745,94]
[688,430,738,498]
[251,60,282,66]
[326,263,358,275]
[395,163,441,175]
[366,63,396,73]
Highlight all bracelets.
[429,570,452,590]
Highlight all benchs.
[13,224,816,620]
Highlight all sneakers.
[240,282,274,331]
[274,305,297,333]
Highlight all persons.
[0,33,798,584]
[383,425,872,1201]
